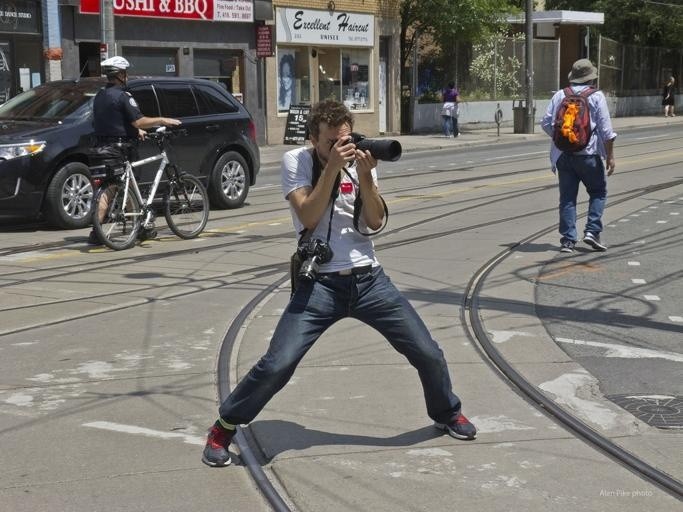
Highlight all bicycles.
[88,127,210,252]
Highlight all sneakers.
[203,422,234,467]
[136,224,157,239]
[434,413,477,439]
[583,232,607,250]
[89,231,112,243]
[560,241,574,253]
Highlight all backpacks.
[553,86,599,152]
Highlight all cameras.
[334,133,402,162]
[296,239,332,285]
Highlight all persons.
[279,54,296,108]
[439,81,460,138]
[87,55,182,246]
[662,76,676,117]
[203,99,478,468]
[541,58,617,253]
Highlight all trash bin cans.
[512,97,537,133]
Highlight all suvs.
[0,76,262,228]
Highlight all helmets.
[100,56,130,75]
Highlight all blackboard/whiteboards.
[284,104,312,145]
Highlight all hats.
[568,59,598,83]
